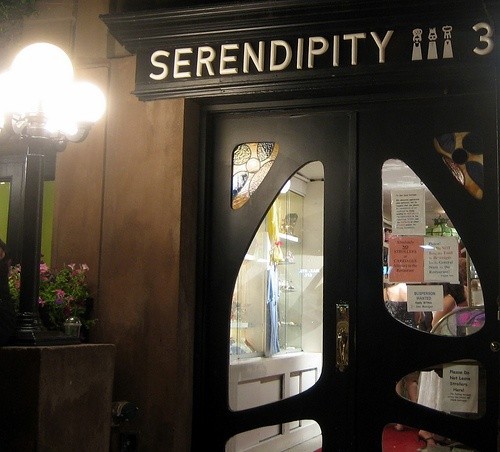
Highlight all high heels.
[417,434,434,447]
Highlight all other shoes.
[395,423,406,431]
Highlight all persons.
[419,278,471,449]
[384,282,424,433]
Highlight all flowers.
[8,263,98,329]
